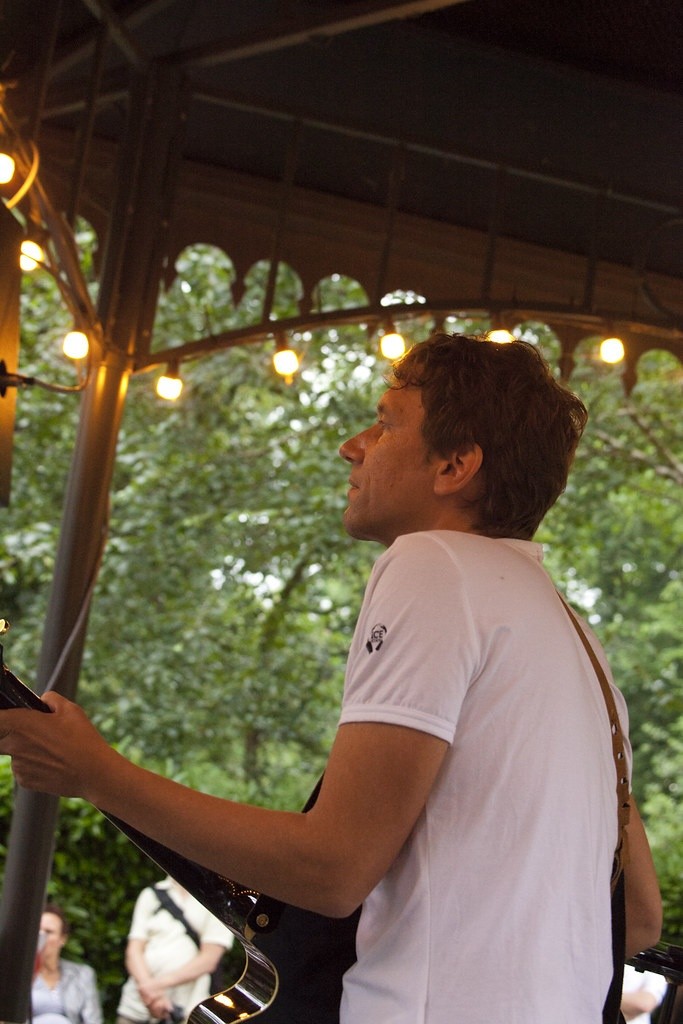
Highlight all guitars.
[0,615,365,1024]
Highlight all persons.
[114,875,234,1024]
[0,332,663,1024]
[32,904,105,1024]
[620,963,668,1024]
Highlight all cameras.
[169,1005,185,1024]
